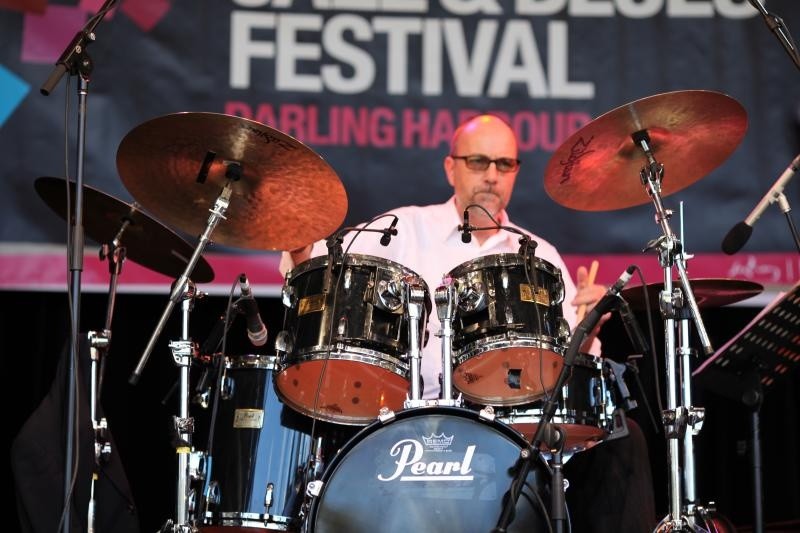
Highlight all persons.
[279,114,612,483]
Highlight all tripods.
[630,129,735,533]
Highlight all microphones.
[240,273,267,345]
[578,264,637,334]
[379,217,398,246]
[461,211,471,243]
[720,155,800,256]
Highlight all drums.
[273,253,432,427]
[189,353,323,533]
[442,251,574,411]
[459,350,609,450]
[299,404,572,533]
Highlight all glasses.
[452,154,521,173]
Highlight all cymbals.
[611,277,766,313]
[116,110,348,252]
[546,90,749,212]
[33,175,216,284]
